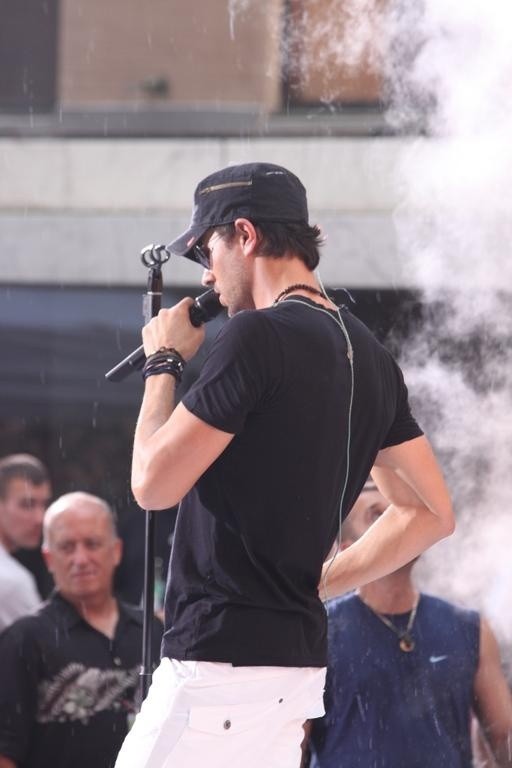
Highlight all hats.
[164,162,309,257]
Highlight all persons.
[0,450,53,632]
[0,486,168,766]
[113,163,459,766]
[300,478,512,766]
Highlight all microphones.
[104,288,224,384]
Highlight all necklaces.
[274,281,326,301]
[363,589,422,651]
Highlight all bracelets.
[139,345,186,382]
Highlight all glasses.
[190,232,242,270]
